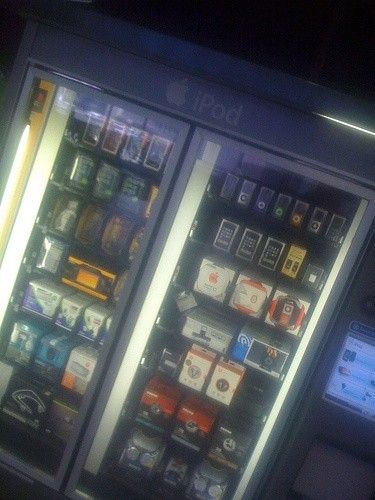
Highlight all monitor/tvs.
[321,332,375,422]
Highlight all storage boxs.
[1,254,312,500]
